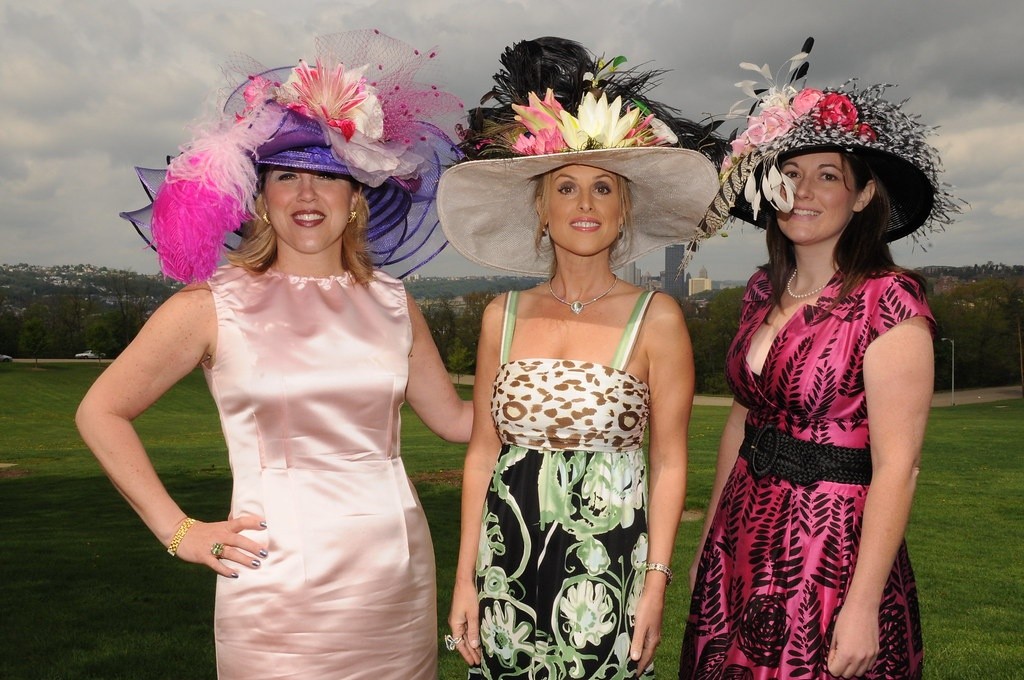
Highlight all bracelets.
[168,518,196,556]
[646,563,672,586]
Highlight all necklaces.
[788,269,826,298]
[549,274,618,314]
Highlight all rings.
[444,635,463,650]
[212,543,225,558]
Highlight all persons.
[436,36,729,680]
[75,29,476,680]
[678,36,937,680]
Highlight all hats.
[120,29,467,279]
[710,36,957,242]
[435,38,718,278]
